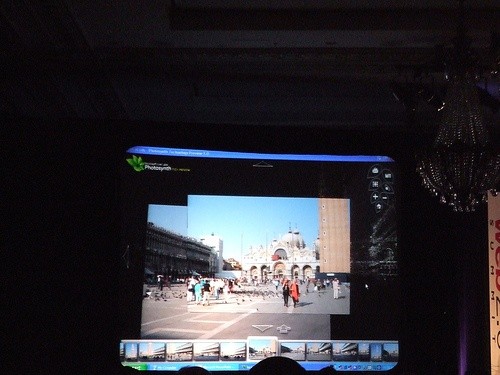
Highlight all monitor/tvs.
[117,144,401,372]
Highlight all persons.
[143,205,350,316]
